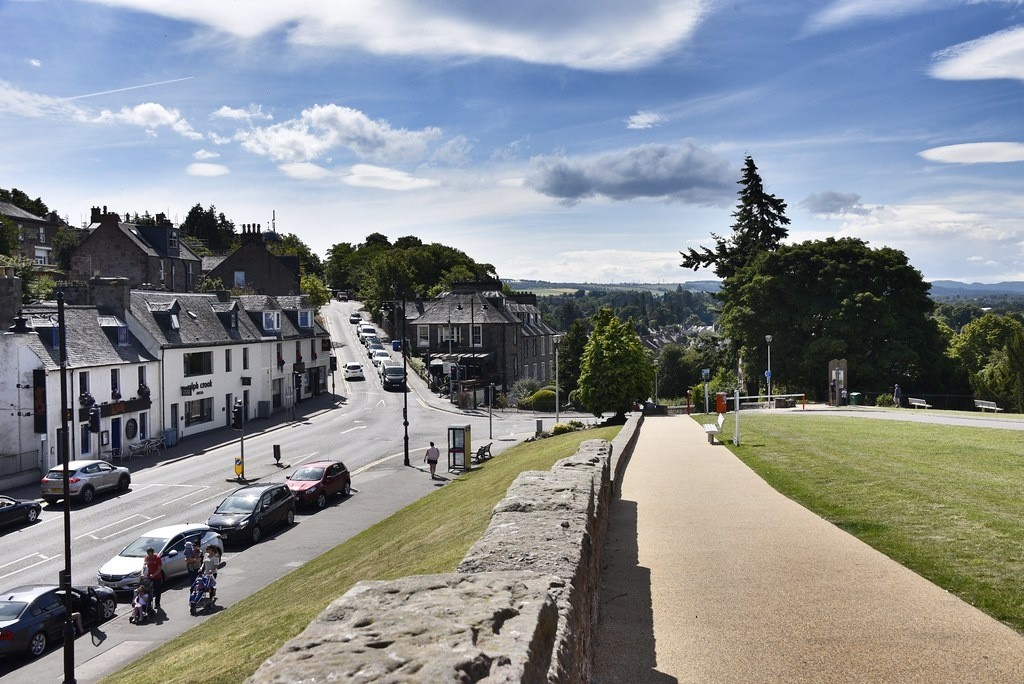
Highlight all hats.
[196,578,202,584]
[194,540,199,545]
[184,542,192,547]
[147,548,154,553]
[138,585,145,592]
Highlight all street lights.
[552,334,563,424]
[765,335,773,409]
[377,291,426,466]
[653,359,659,408]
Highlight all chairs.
[129,436,167,459]
[102,447,123,465]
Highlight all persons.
[132,539,219,622]
[841,387,847,406]
[72,612,84,633]
[830,379,836,405]
[893,384,901,408]
[424,441,440,479]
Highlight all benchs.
[704,413,725,445]
[973,399,1004,413]
[470,441,493,464]
[908,398,933,409]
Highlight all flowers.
[137,382,151,396]
[79,392,95,406]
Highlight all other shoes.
[191,601,196,603]
[154,604,160,608]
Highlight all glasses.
[193,545,198,547]
[206,549,209,550]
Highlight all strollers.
[189,570,217,616]
[128,573,154,624]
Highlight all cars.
[349,312,408,392]
[0,581,118,662]
[39,459,132,507]
[342,361,364,381]
[350,312,363,324]
[96,522,224,601]
[336,291,348,302]
[0,494,42,531]
[206,482,297,546]
[284,459,351,511]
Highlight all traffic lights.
[450,365,458,381]
[232,404,242,429]
[88,408,101,433]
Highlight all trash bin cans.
[168,428,177,447]
[537,420,542,433]
[161,430,172,448]
[258,401,270,418]
[849,392,861,405]
